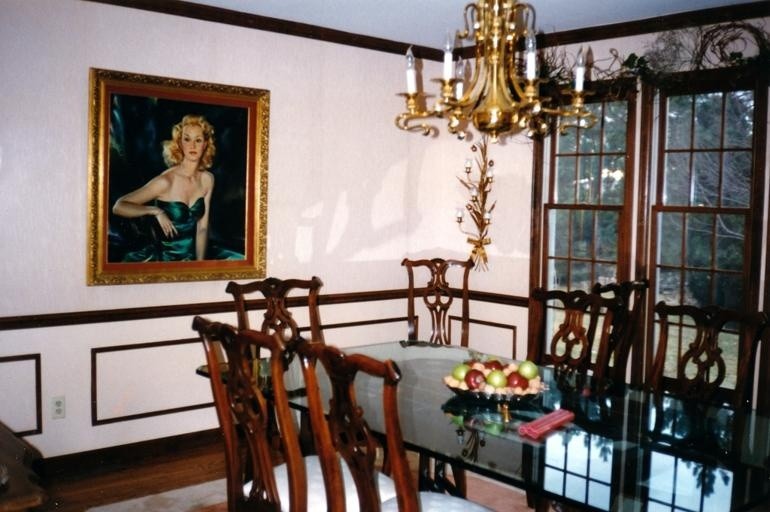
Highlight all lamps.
[456,135,501,271]
[393,0,596,141]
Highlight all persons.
[111,114,245,262]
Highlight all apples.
[451,355,539,390]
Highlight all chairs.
[588,281,651,368]
[401,258,475,499]
[195,319,396,512]
[226,277,325,354]
[287,337,487,512]
[644,302,765,412]
[528,288,623,372]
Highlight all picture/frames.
[86,67,267,284]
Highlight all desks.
[1,421,47,511]
